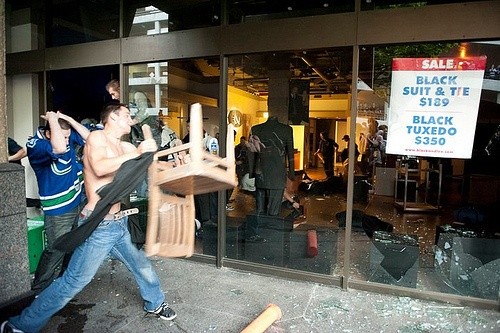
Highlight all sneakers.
[144,302,177,321]
[1,320,24,333]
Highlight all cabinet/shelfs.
[467,174,500,206]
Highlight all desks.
[392,164,443,214]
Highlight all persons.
[0,80,250,333]
[358,119,389,178]
[314,131,360,179]
[249,115,297,218]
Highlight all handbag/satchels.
[453,205,484,225]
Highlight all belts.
[81,208,139,220]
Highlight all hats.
[342,134,349,139]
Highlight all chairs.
[141,103,238,259]
